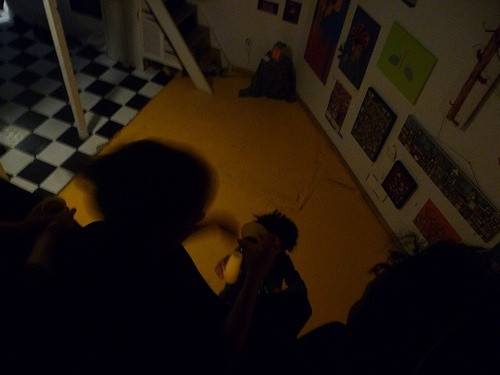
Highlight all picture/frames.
[352,88,397,161]
[383,159,417,209]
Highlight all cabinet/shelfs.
[142,11,182,71]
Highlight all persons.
[237,42,298,103]
[1,139,500,374]
